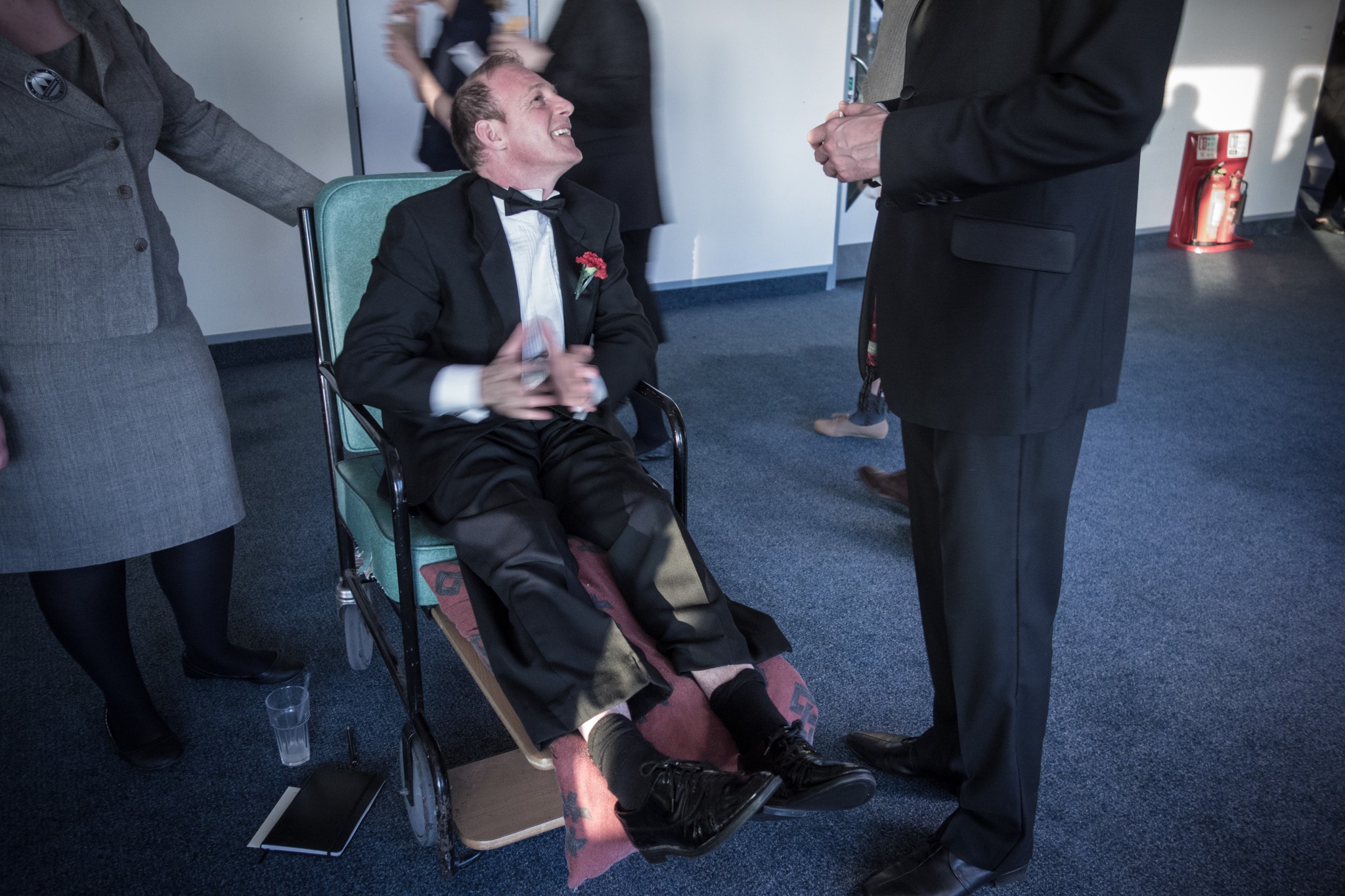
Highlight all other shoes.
[104,705,184,770]
[182,649,305,684]
[814,413,889,439]
[858,465,910,506]
[1312,218,1345,235]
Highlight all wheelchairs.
[295,169,689,877]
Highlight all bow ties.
[487,179,566,219]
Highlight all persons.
[0,0,327,770]
[324,54,878,862]
[807,0,1186,895]
[387,1,669,457]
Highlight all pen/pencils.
[347,725,359,765]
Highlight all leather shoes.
[614,758,783,864]
[859,841,1029,896]
[847,731,922,779]
[737,719,876,821]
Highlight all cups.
[264,685,311,767]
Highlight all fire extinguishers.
[1192,163,1227,246]
[1217,170,1248,244]
